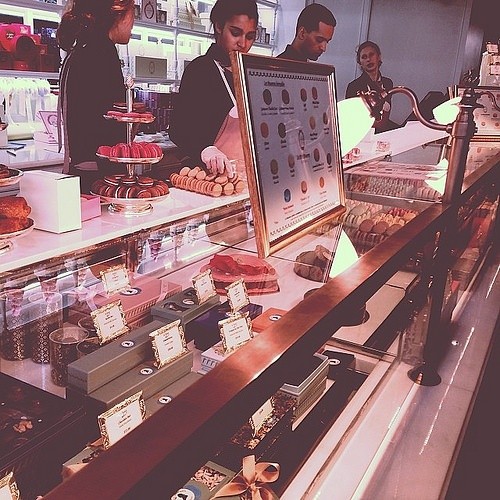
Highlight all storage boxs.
[19,170,82,234]
[1,286,354,500]
[67,271,182,323]
[81,194,101,222]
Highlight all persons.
[168,0,259,179]
[345,41,393,134]
[276,3,336,63]
[56,0,135,195]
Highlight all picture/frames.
[229,50,348,259]
[454,84,500,142]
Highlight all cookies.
[293,244,332,282]
[331,177,440,249]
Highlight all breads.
[0,196,32,235]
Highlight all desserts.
[105,102,152,119]
[90,174,168,199]
[170,167,244,197]
[96,140,162,158]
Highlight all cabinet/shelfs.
[0,1,281,171]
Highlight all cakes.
[199,254,279,295]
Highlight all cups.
[1,289,109,388]
